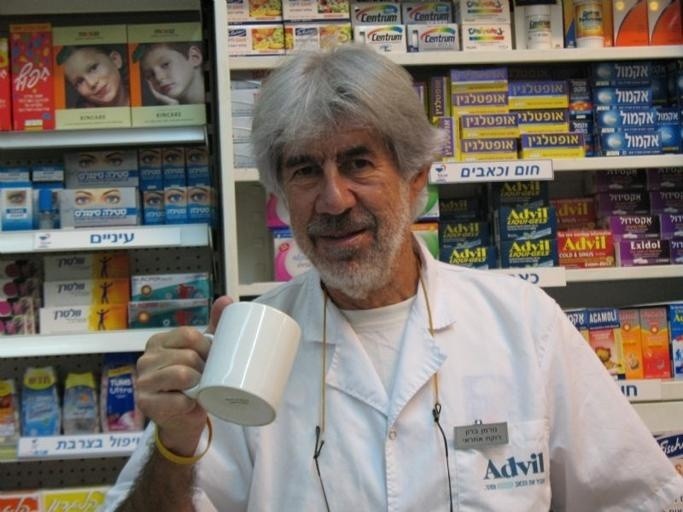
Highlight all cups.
[179,301,300,426]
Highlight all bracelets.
[155,416,212,465]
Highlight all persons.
[8,190,27,204]
[100,45,682,511]
[143,147,210,207]
[75,152,123,207]
[56,43,129,107]
[132,40,205,107]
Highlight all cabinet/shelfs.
[0,1,681,510]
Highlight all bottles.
[526,2,604,50]
